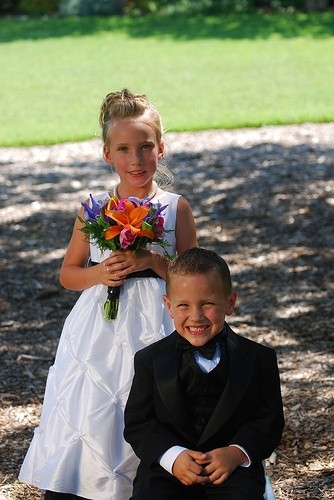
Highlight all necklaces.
[113,179,158,201]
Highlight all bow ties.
[175,329,225,361]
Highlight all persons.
[122,246,287,500]
[17,87,201,500]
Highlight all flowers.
[78,187,167,321]
